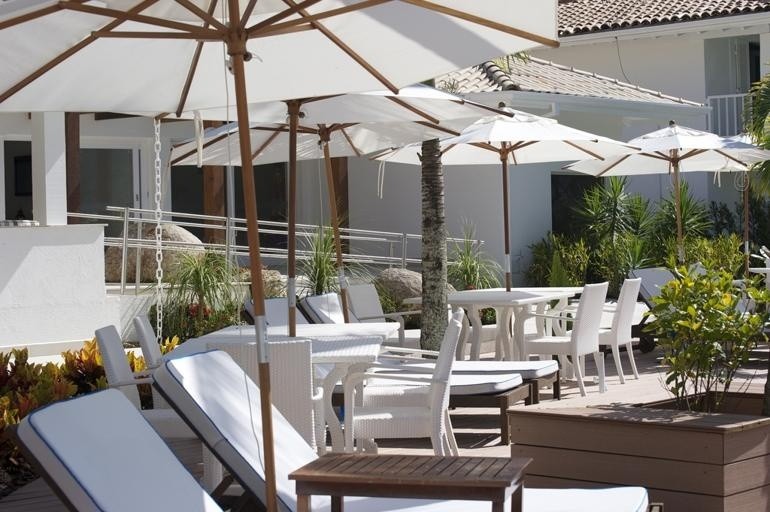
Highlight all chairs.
[202,339,327,484]
[400,258,768,399]
[243,283,540,453]
[18,387,223,511]
[95,324,163,410]
[146,349,650,511]
[129,315,163,367]
